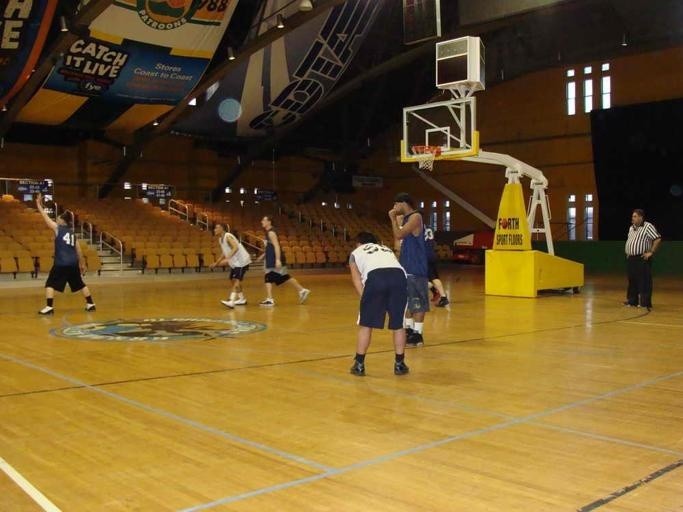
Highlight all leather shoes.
[394,362,409,374]
[351,362,365,376]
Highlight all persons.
[255,215,311,308]
[35,192,96,316]
[348,193,450,375]
[622,209,662,311]
[209,223,252,309]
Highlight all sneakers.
[260,298,275,306]
[38,305,55,315]
[623,301,655,308]
[437,299,449,306]
[234,297,248,305]
[431,285,442,300]
[406,329,424,347]
[221,297,235,309]
[298,289,311,304]
[85,303,96,311]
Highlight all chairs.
[1,192,454,272]
